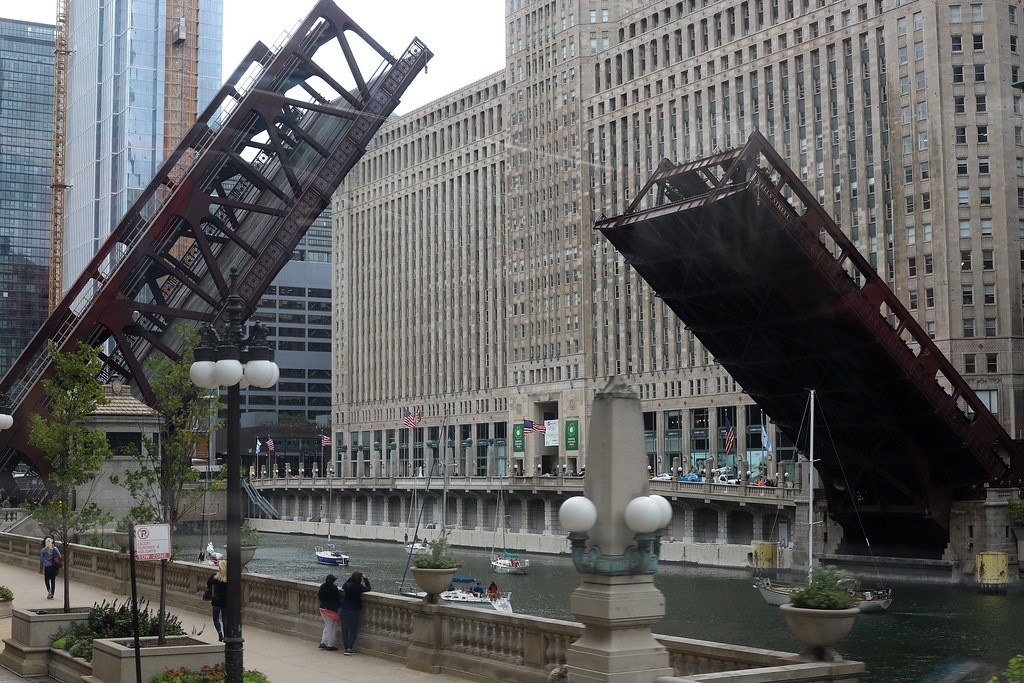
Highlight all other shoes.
[50,596,54,600]
[47,593,52,599]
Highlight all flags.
[760,425,773,458]
[255,439,262,454]
[724,416,735,456]
[403,407,416,429]
[265,438,275,450]
[414,406,422,424]
[524,419,546,435]
[322,434,332,446]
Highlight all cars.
[649,472,776,486]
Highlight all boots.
[219,631,224,642]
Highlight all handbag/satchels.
[52,544,63,568]
[202,574,219,601]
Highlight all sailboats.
[753,389,892,613]
[489,474,529,574]
[405,476,446,556]
[314,478,349,566]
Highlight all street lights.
[513,464,567,476]
[647,465,789,483]
[190,263,281,683]
[248,461,333,478]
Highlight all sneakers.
[344,648,356,655]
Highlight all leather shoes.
[319,643,327,648]
[326,647,338,651]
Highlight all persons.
[496,554,522,569]
[205,559,227,641]
[423,538,427,546]
[340,571,371,655]
[475,582,481,592]
[317,574,343,650]
[488,581,498,598]
[39,538,62,600]
[41,528,56,545]
[448,583,455,591]
[404,533,408,544]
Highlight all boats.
[395,576,513,613]
[203,551,227,566]
